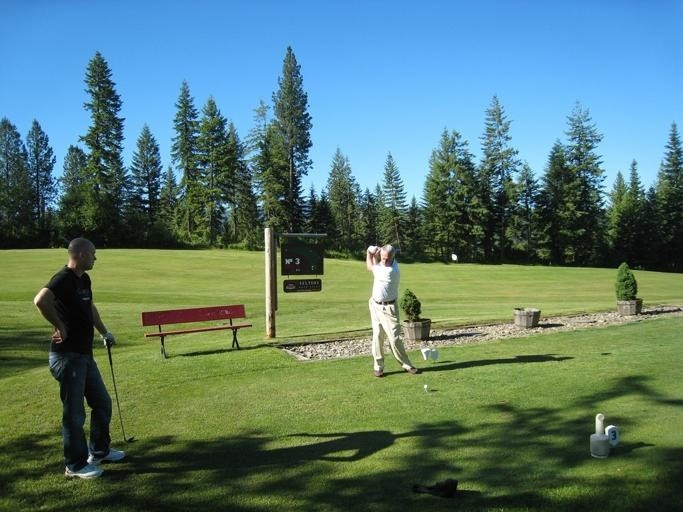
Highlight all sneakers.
[65,463,104,480]
[405,367,417,374]
[87,448,126,465]
[374,370,383,377]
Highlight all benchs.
[141,304,253,359]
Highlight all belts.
[373,298,394,305]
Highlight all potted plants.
[398,289,430,340]
[614,262,643,316]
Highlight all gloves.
[99,333,117,349]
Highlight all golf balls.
[424,385,427,388]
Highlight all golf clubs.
[106,340,134,444]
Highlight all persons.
[366,244,418,377]
[33,238,125,481]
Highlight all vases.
[512,307,541,328]
